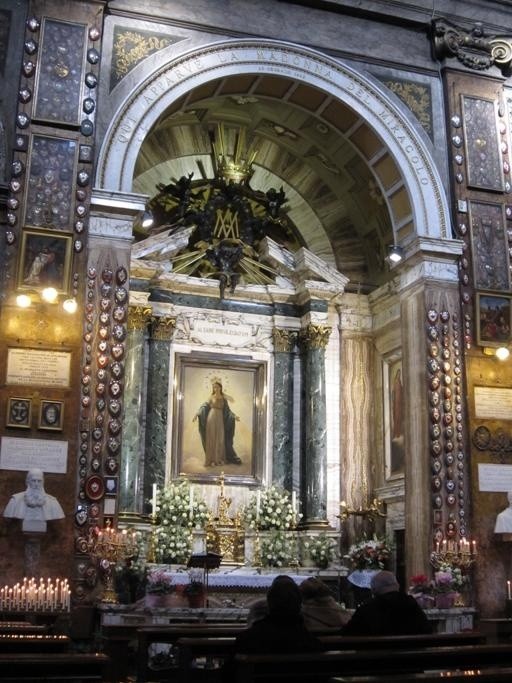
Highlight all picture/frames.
[475,292,512,347]
[37,398,65,431]
[382,349,407,482]
[171,351,268,486]
[15,226,74,297]
[5,397,32,428]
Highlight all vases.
[416,594,472,608]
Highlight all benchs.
[0,615,512,683]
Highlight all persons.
[482,304,511,340]
[162,170,295,301]
[3,468,65,522]
[23,245,64,288]
[243,575,354,632]
[221,573,326,682]
[393,368,403,438]
[191,379,242,466]
[345,568,433,674]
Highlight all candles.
[188,487,193,522]
[256,490,260,519]
[1,576,71,612]
[292,490,296,511]
[152,483,156,511]
[507,580,511,600]
[437,537,477,555]
[91,527,141,546]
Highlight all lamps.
[16,287,79,317]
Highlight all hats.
[370,571,400,597]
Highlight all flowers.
[347,536,394,570]
[244,481,304,530]
[155,480,209,529]
[411,564,469,594]
[256,530,334,567]
[147,526,191,567]
